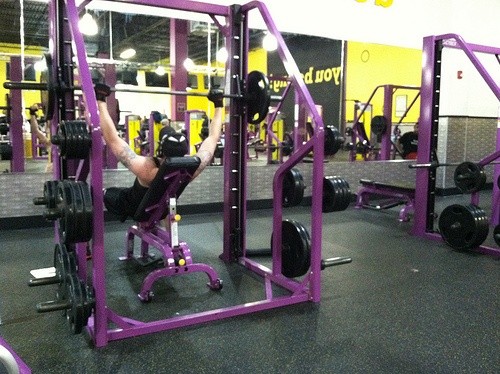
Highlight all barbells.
[370,116,420,133]
[4,52,283,126]
[0,96,133,125]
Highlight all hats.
[159,127,188,158]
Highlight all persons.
[92,82,225,222]
[29,105,52,148]
[396,121,420,161]
